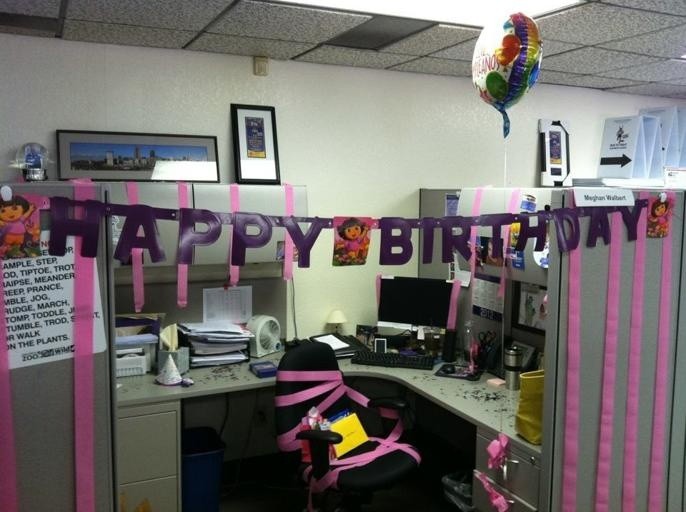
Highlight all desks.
[115,360,539,512]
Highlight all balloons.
[471,13,544,140]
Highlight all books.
[177,319,255,368]
[310,332,370,359]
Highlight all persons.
[335,218,371,261]
[647,199,672,235]
[536,305,546,328]
[0,196,37,258]
[524,291,535,327]
[115,313,168,372]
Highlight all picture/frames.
[228,102,282,186]
[57,127,222,184]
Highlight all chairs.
[272,339,436,512]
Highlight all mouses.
[442,365,455,374]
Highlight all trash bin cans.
[442,469,474,512]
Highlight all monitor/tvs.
[375,273,461,355]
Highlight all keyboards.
[351,351,433,370]
